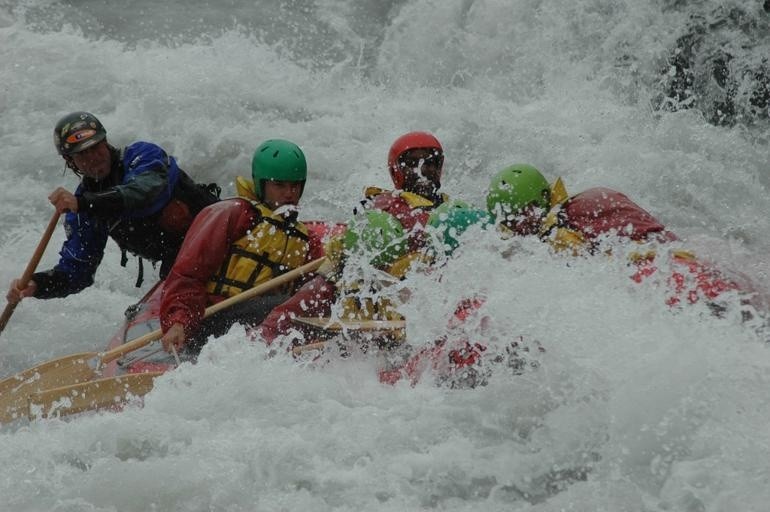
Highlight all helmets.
[388,131,443,189]
[54,112,106,155]
[487,163,550,225]
[251,139,307,198]
[426,198,494,255]
[342,209,408,267]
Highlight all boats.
[102,218,770,407]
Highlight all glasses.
[404,154,438,168]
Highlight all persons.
[7,111,221,304]
[355,129,448,252]
[486,162,665,272]
[160,139,312,354]
[246,209,410,358]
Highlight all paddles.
[27,342,327,424]
[0,204,71,335]
[292,314,407,333]
[0,257,327,423]
